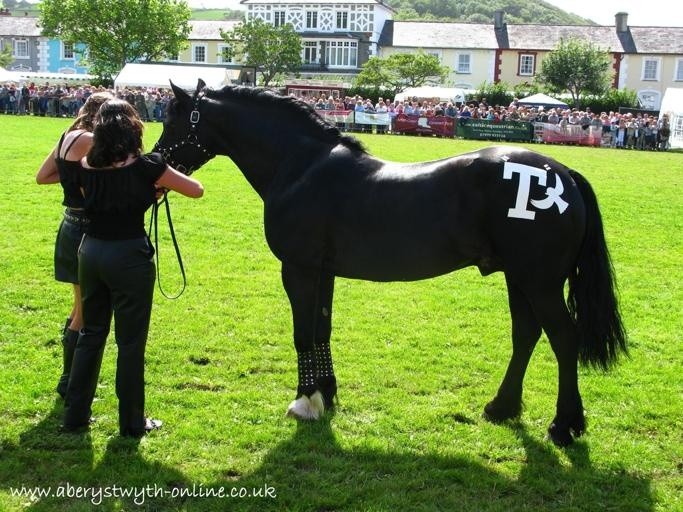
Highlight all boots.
[57,318,78,399]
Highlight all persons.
[0,78,673,154]
[31,90,117,402]
[53,95,206,435]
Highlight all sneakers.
[145,418,162,431]
[63,417,95,432]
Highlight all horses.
[149,78,635,447]
